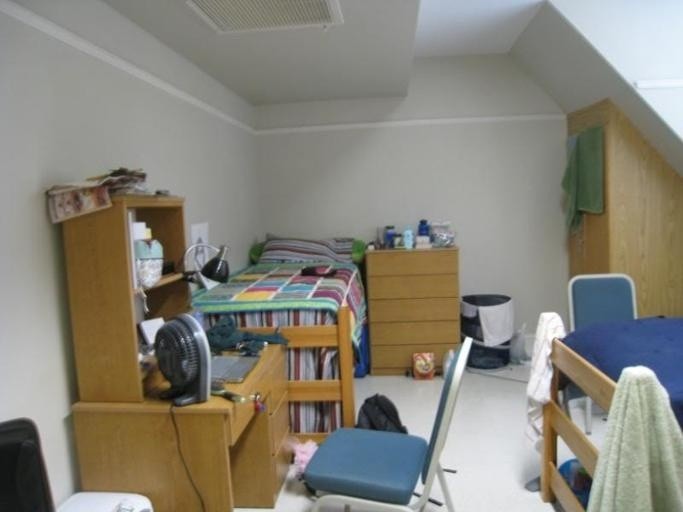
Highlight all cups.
[402,229,414,250]
[415,236,432,249]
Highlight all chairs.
[540,274,663,417]
[0,417,153,512]
[304,337,473,512]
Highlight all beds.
[195,236,369,445]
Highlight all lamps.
[170,228,241,300]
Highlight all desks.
[71,341,296,512]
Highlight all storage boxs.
[131,239,164,287]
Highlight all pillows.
[248,232,365,278]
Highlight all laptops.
[191,310,260,383]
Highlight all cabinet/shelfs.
[42,190,191,402]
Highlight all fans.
[154,313,213,406]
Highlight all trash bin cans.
[460,294,514,369]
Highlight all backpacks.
[356,392,407,436]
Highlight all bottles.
[383,225,396,249]
[417,219,429,235]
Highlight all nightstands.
[354,241,470,374]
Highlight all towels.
[478,297,516,349]
[460,297,477,318]
[524,309,574,436]
[587,344,683,512]
[561,129,604,230]
